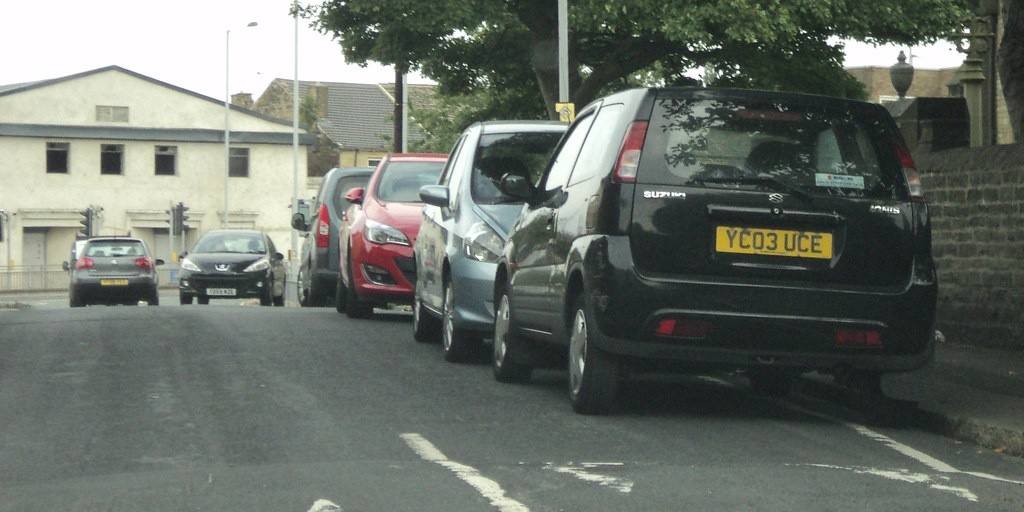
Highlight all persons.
[214,240,225,252]
[248,240,258,251]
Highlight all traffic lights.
[178,202,190,235]
[78,208,93,238]
[165,209,176,236]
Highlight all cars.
[290,163,439,307]
[488,82,937,418]
[334,152,451,320]
[176,230,286,307]
[412,118,576,368]
[61,237,161,307]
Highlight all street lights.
[223,19,260,229]
